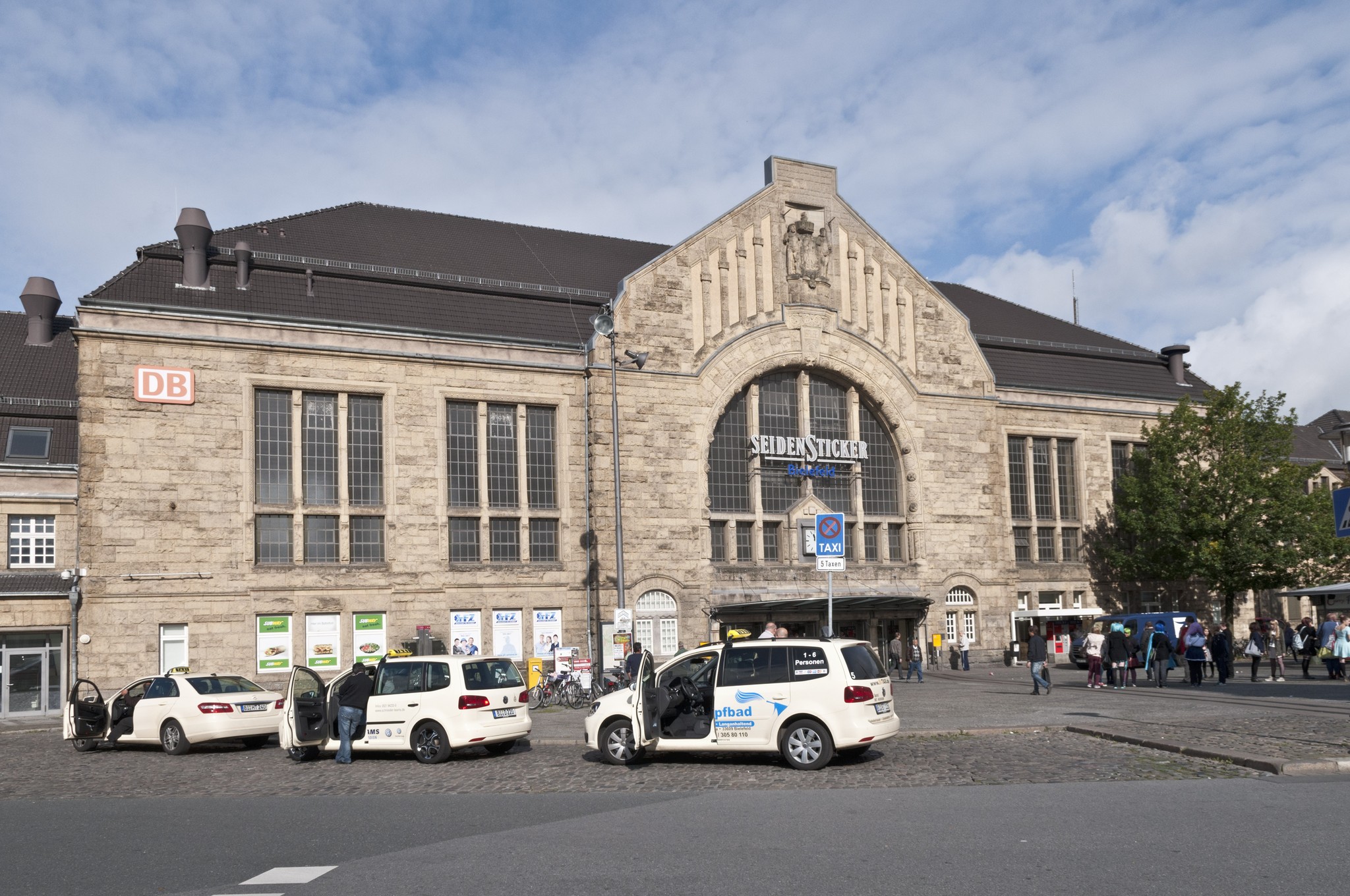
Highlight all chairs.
[466,668,481,687]
[391,673,406,693]
[730,660,756,686]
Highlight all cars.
[64,667,285,756]
[278,654,534,763]
[584,636,901,772]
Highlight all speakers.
[78,634,91,644]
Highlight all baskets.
[558,675,569,680]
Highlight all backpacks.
[1291,626,1309,650]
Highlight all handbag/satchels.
[1102,653,1112,671]
[1314,622,1325,647]
[1078,639,1089,658]
[1041,663,1051,684]
[1325,629,1335,650]
[1149,647,1156,661]
[1244,635,1262,657]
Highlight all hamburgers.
[314,644,333,655]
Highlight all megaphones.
[624,349,650,371]
[593,314,615,336]
[588,304,607,326]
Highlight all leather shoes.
[100,740,116,747]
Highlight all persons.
[1107,623,1131,689]
[1084,627,1108,689]
[1202,628,1214,678]
[1295,617,1317,679]
[536,634,547,652]
[1140,622,1154,681]
[1155,620,1166,626]
[1209,623,1229,686]
[624,642,632,659]
[773,627,789,638]
[674,640,691,670]
[1026,625,1053,695]
[1266,620,1286,681]
[460,638,471,654]
[1177,616,1195,682]
[1283,622,1295,655]
[1219,623,1235,679]
[626,642,649,683]
[1249,622,1264,682]
[1123,627,1140,687]
[1333,615,1350,679]
[1099,624,1114,686]
[956,631,970,671]
[453,638,465,654]
[99,682,155,747]
[906,639,924,683]
[1317,614,1342,680]
[335,662,373,764]
[550,634,559,651]
[1183,623,1207,687]
[757,622,777,639]
[542,636,553,652]
[887,632,905,680]
[1152,623,1172,688]
[466,638,478,655]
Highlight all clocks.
[801,527,816,556]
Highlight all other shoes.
[1251,677,1262,682]
[1276,677,1285,681]
[960,669,963,671]
[337,760,351,764]
[1047,683,1052,694]
[906,680,909,684]
[1303,674,1315,679]
[918,680,924,684]
[1087,673,1234,688]
[1030,690,1040,695]
[1329,673,1350,682]
[1265,675,1275,681]
[899,677,906,680]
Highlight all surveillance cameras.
[59,572,71,583]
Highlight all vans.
[1069,612,1198,668]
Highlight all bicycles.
[527,663,626,709]
[1231,636,1303,661]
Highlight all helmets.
[460,638,467,643]
